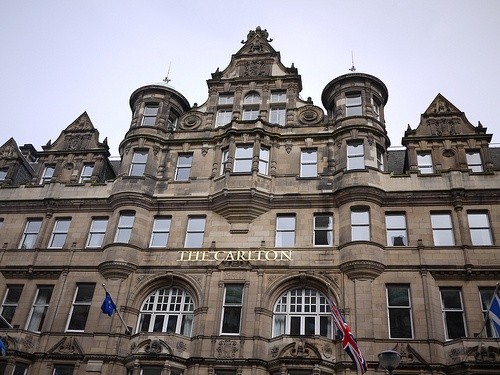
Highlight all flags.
[324,297,368,375]
[487,294,499,329]
[99,294,117,317]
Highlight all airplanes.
[162,77,171,83]
[349,66,356,71]
